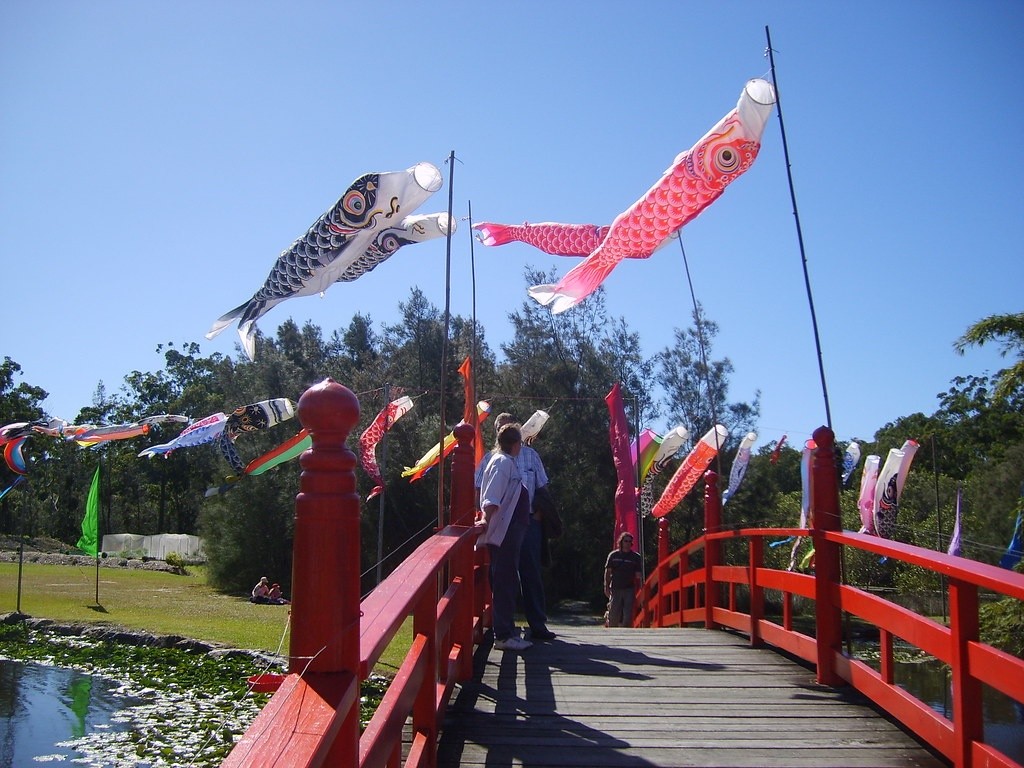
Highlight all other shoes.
[527,627,556,639]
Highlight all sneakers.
[493,637,534,651]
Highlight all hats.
[261,577,268,583]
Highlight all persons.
[602,532,642,628]
[474,412,557,639]
[252,577,271,604]
[269,583,291,605]
[472,423,533,650]
[604,603,611,627]
